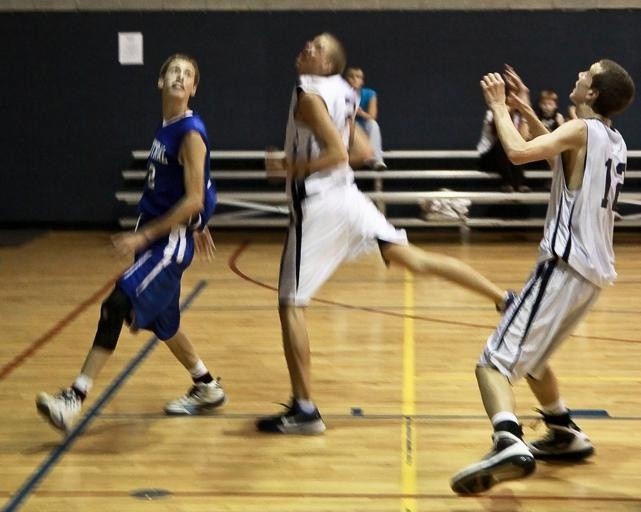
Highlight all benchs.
[117,150,641,226]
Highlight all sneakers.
[450,432,535,494]
[528,407,594,461]
[36,384,82,436]
[164,376,225,415]
[256,398,327,434]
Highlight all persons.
[345,64,388,170]
[35,52,227,438]
[449,59,635,496]
[475,90,579,192]
[256,33,518,433]
